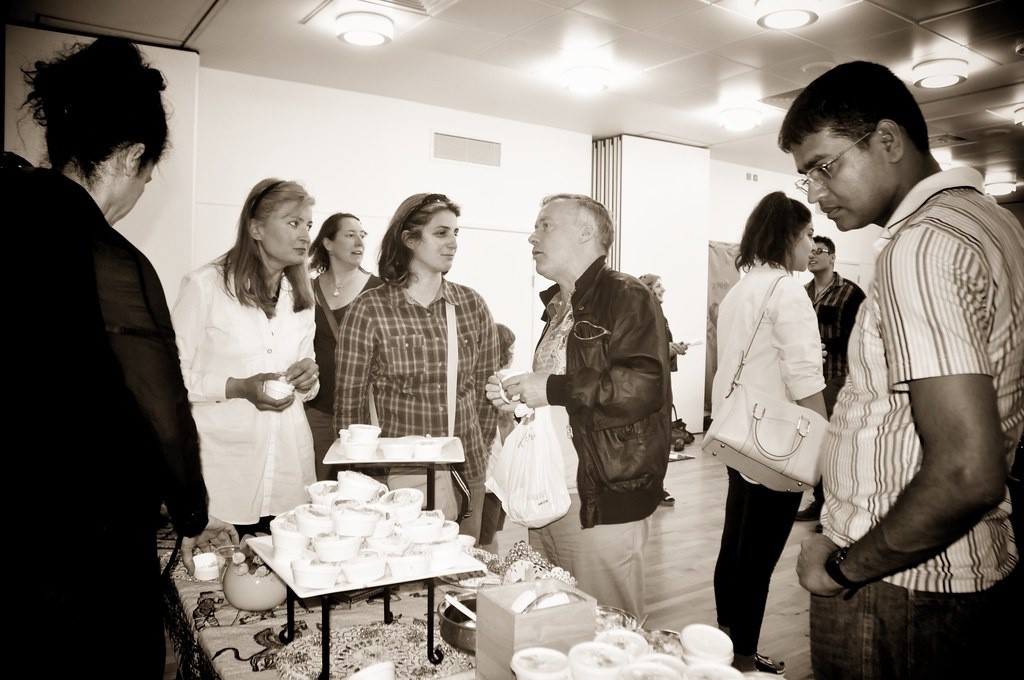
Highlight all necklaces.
[329,267,357,297]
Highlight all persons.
[777,60,1024,680]
[332,192,500,545]
[475,324,516,562]
[169,179,320,541]
[638,274,690,508]
[794,237,867,531]
[485,198,673,632]
[711,191,829,678]
[302,212,384,481]
[0,30,225,680]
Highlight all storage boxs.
[475,578,597,680]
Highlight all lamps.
[335,11,394,46]
[912,59,968,89]
[756,0,819,30]
[983,172,1019,195]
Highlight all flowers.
[231,546,272,576]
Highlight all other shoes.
[796,502,821,521]
[661,490,676,505]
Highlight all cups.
[346,661,396,680]
[510,624,787,680]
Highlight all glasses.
[814,248,832,255]
[407,195,451,221]
[796,130,874,194]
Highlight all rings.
[312,375,317,380]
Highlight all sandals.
[756,652,786,674]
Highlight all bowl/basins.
[265,379,295,401]
[338,423,441,461]
[192,553,226,581]
[438,592,476,653]
[269,469,459,590]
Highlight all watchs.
[826,548,866,601]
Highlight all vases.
[222,561,287,611]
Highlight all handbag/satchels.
[700,380,831,491]
[388,465,458,521]
[484,373,572,529]
[671,403,695,444]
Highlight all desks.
[160,545,496,680]
